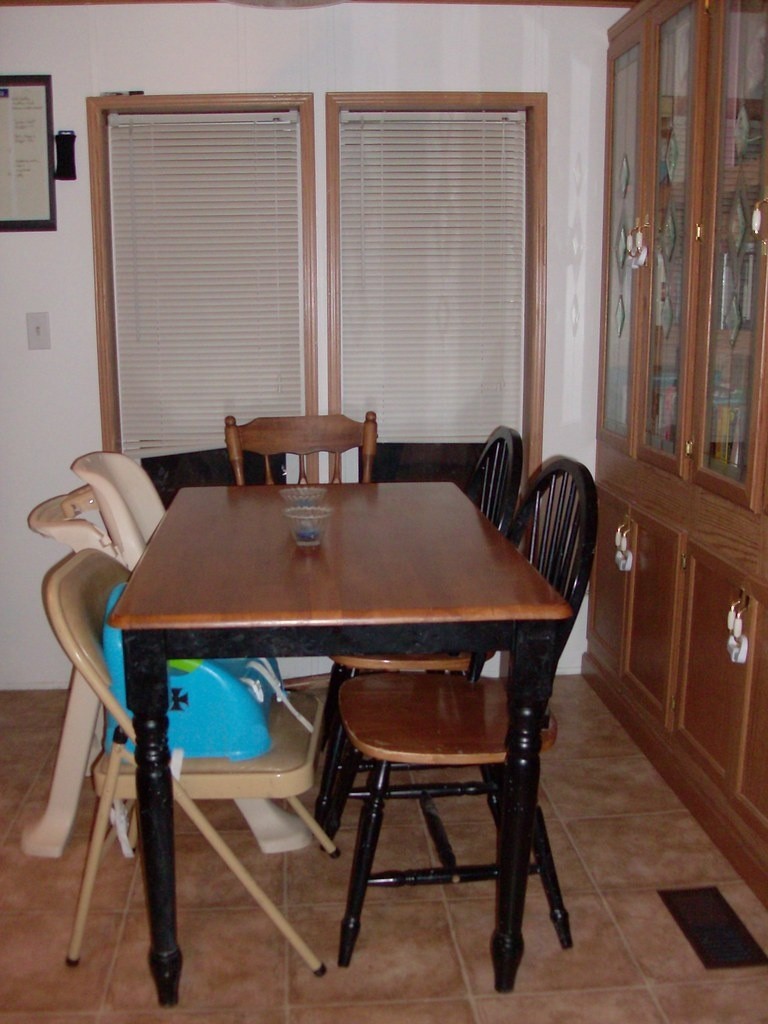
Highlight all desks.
[110,482,573,1010]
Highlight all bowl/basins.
[279,487,327,508]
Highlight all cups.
[281,506,332,546]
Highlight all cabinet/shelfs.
[581,1,768,915]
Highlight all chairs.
[19,411,598,973]
